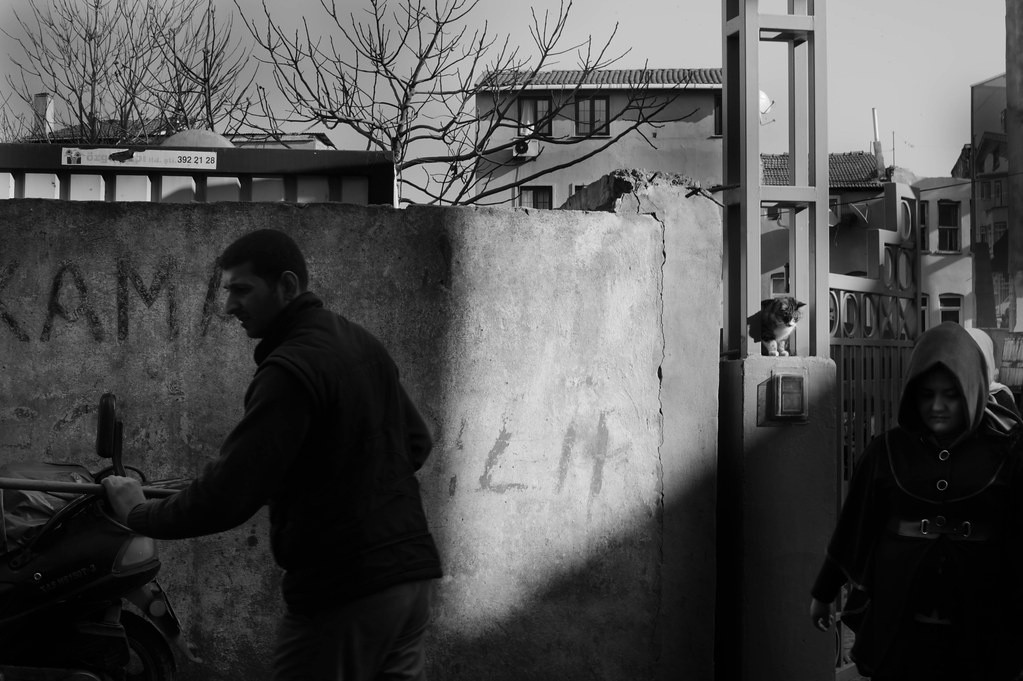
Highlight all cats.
[761,296,807,356]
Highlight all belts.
[889,514,993,541]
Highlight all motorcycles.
[0,394,204,681]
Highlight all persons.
[100,229,441,681]
[809,320,1023,681]
